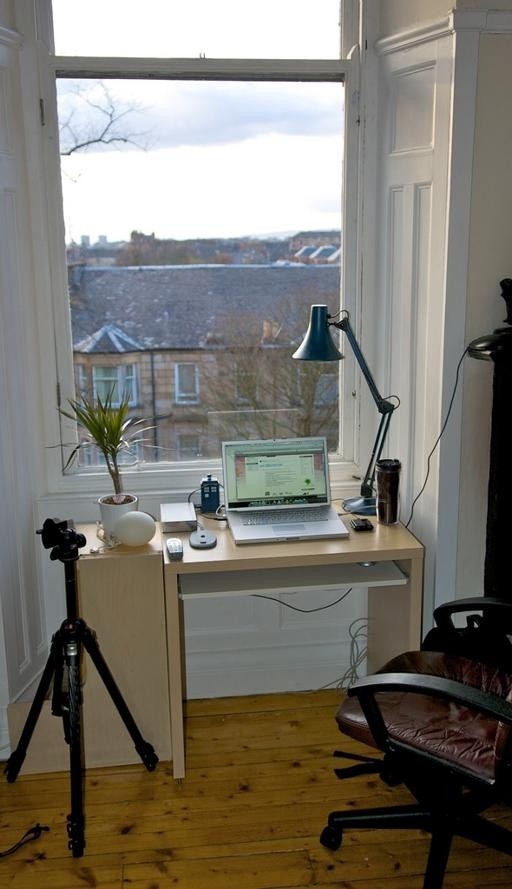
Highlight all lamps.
[292,305,396,514]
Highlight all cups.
[374,458,402,526]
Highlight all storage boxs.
[6,698,70,776]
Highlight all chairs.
[320,598,512,888]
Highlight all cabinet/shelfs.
[72,523,171,770]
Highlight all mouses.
[358,561,377,567]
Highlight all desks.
[178,560,409,701]
[165,500,424,779]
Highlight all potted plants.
[44,381,177,549]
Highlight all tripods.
[3,518,159,857]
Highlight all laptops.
[221,436,349,544]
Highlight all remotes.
[166,538,183,560]
